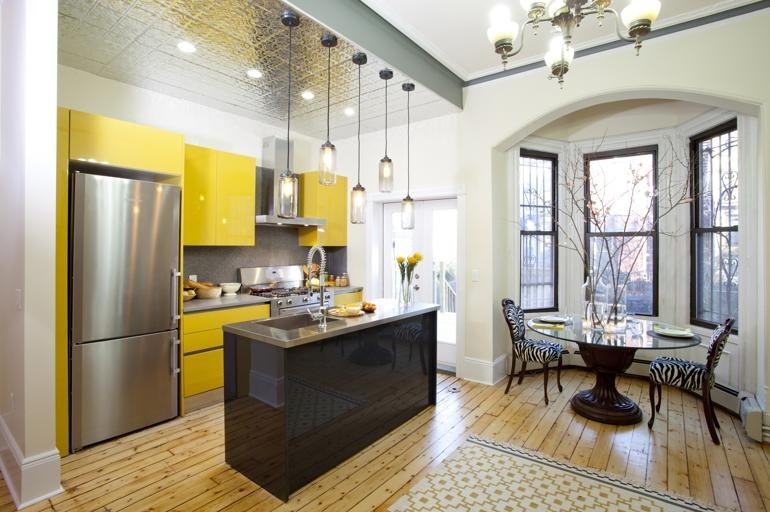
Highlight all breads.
[363,303,376,312]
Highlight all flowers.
[528,127,627,330]
[396,251,424,285]
[595,143,712,328]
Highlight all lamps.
[478,0,668,90]
[400,80,417,234]
[348,51,371,226]
[276,9,304,222]
[376,66,397,196]
[317,31,341,189]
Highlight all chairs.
[645,314,736,446]
[501,297,565,409]
[391,312,430,376]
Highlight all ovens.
[271,298,330,318]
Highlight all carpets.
[286,373,367,447]
[381,436,728,512]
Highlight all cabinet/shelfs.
[297,168,348,247]
[332,289,366,306]
[184,143,255,249]
[182,303,272,414]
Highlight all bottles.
[323,271,348,288]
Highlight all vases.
[582,293,606,324]
[399,277,417,309]
[604,301,629,334]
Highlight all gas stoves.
[246,288,331,308]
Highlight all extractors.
[255,135,328,228]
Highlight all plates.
[539,315,566,323]
[657,328,694,338]
[328,307,366,318]
[184,294,198,301]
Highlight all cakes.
[336,303,362,315]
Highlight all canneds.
[324,272,328,287]
[328,275,335,281]
[340,277,347,287]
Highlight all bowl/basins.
[219,282,241,296]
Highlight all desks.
[526,313,702,426]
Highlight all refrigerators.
[70,170,180,453]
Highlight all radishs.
[186,278,206,289]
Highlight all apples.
[183,288,196,296]
